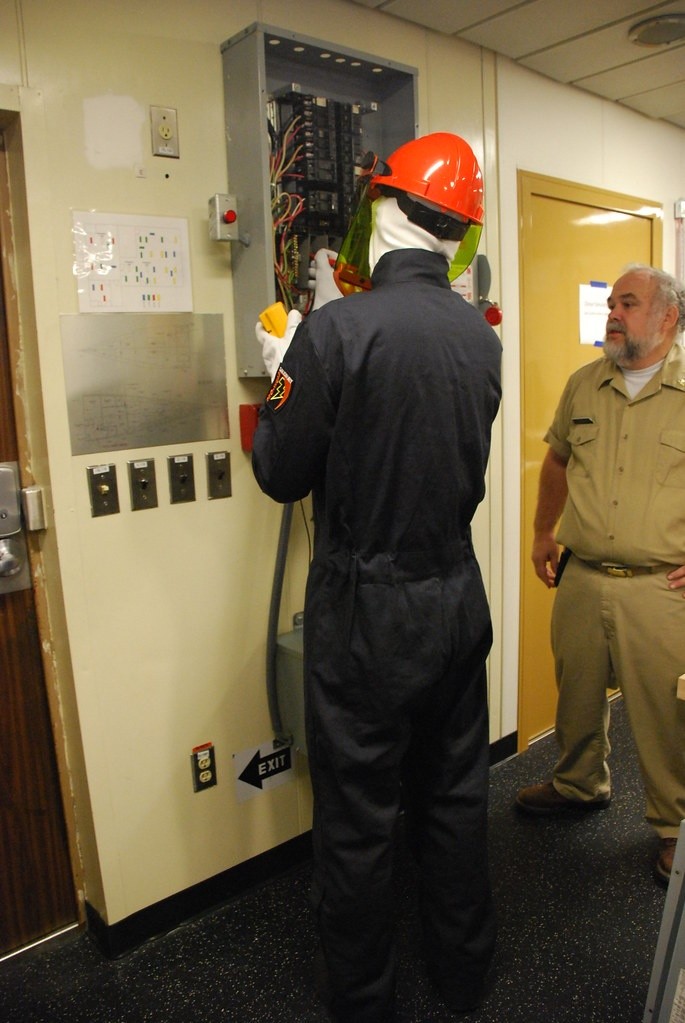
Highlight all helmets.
[371,133,484,240]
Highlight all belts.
[580,560,681,578]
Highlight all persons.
[253,131,507,1023]
[511,266,682,883]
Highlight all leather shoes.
[515,783,610,811]
[656,838,678,882]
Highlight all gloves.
[255,310,302,384]
[308,248,362,312]
